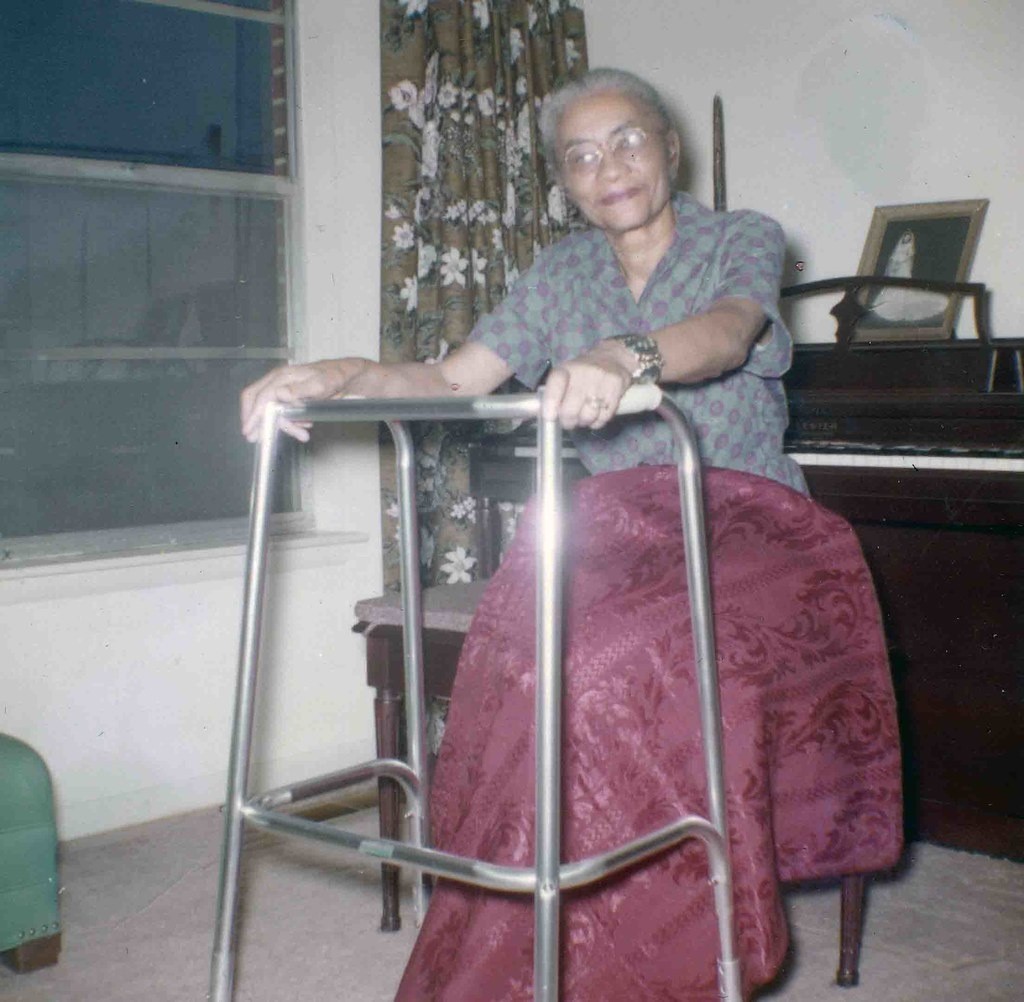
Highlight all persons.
[872,228,950,321]
[237,68,812,1002]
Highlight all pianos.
[466,277,1024,862]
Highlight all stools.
[349,579,494,936]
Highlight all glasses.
[558,127,666,178]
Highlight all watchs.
[605,335,664,386]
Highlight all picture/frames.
[852,198,989,342]
